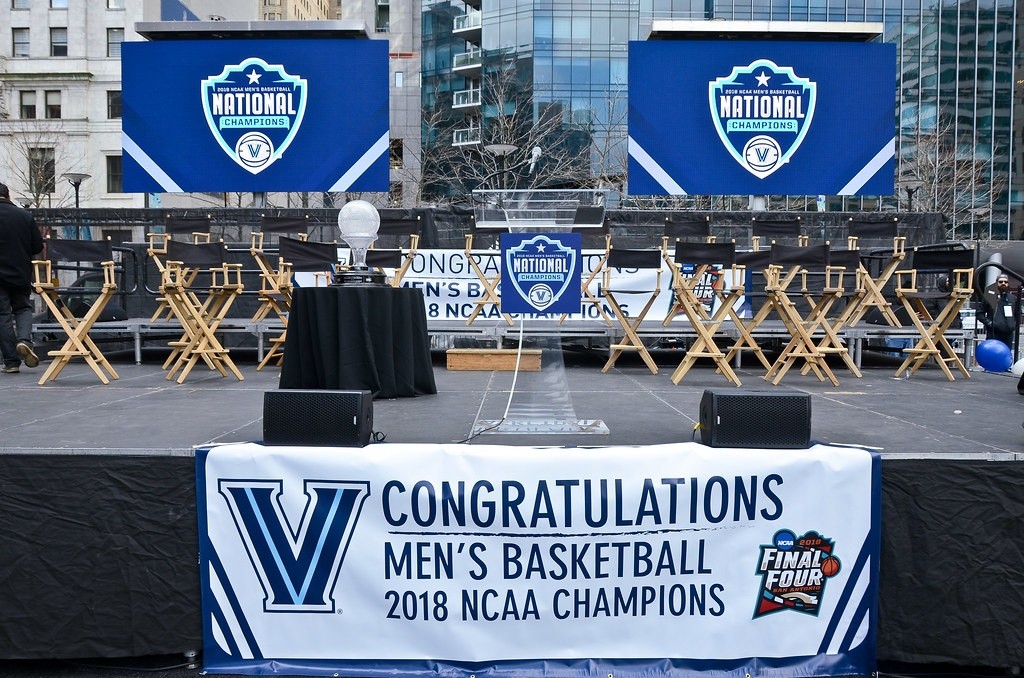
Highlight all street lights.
[967,207,992,269]
[484,143,519,250]
[62,173,92,279]
[897,180,925,213]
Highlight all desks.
[279,286,438,399]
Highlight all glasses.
[997,281,1009,284]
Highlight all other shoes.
[1,365,20,373]
[17,343,39,368]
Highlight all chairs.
[31,215,422,384]
[465,216,515,326]
[568,207,974,387]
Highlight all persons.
[0,183,45,373]
[976,273,1022,372]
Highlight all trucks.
[32,241,299,359]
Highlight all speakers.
[80,298,129,322]
[865,305,913,326]
[699,389,812,449]
[263,388,374,447]
